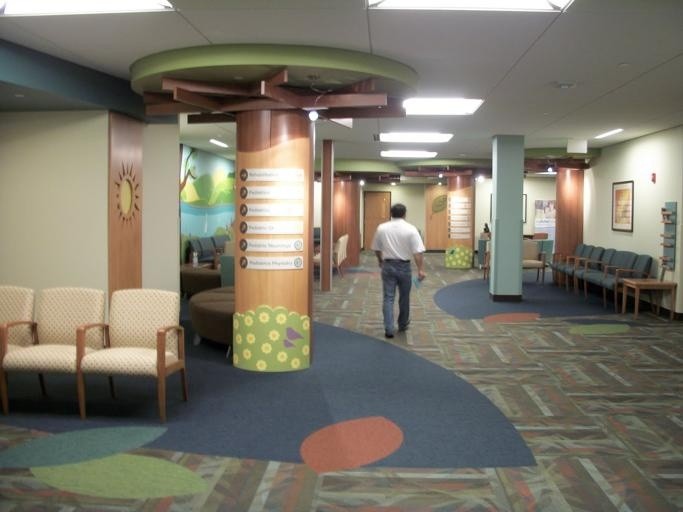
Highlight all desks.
[621,276,679,321]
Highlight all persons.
[370,203,426,338]
[541,206,552,219]
[548,201,556,219]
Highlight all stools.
[180,260,236,357]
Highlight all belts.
[384,258,411,263]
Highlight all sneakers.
[398,324,410,331]
[385,331,395,338]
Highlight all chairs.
[311,227,349,280]
[0,284,190,422]
[186,231,231,261]
[520,231,658,319]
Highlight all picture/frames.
[611,181,635,234]
